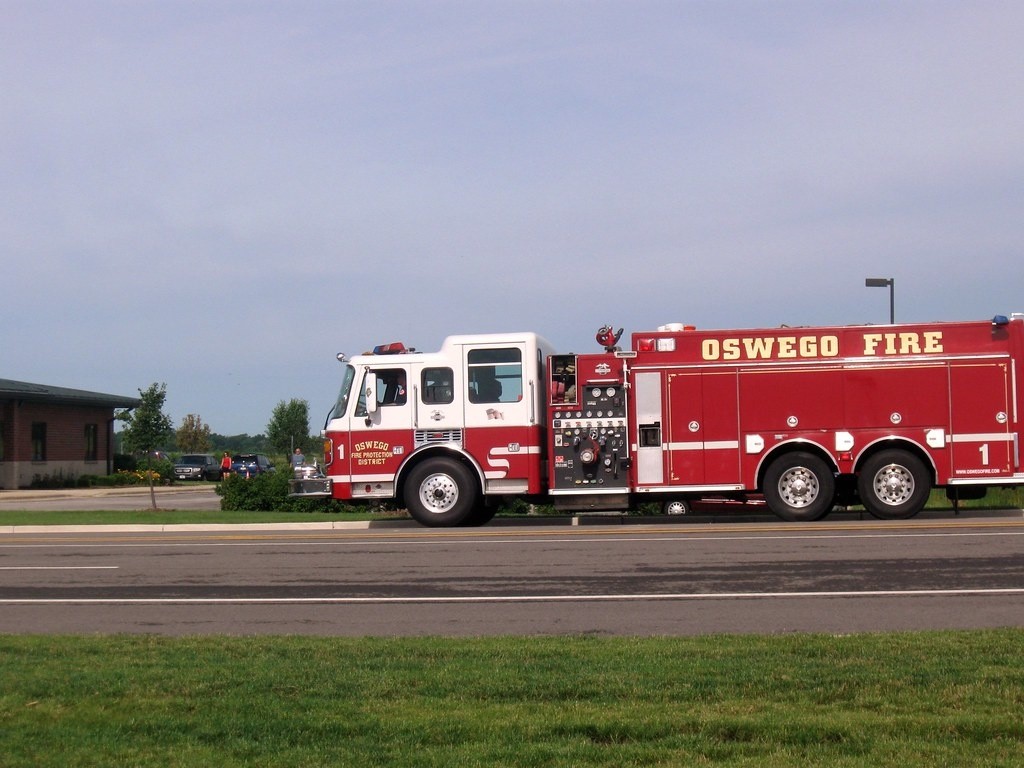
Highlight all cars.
[135,450,175,462]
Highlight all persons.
[377,371,406,406]
[289,448,305,468]
[488,380,502,401]
[220,451,231,482]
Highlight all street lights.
[864,277,896,325]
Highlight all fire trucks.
[286,314,1024,528]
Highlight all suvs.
[172,454,222,482]
[219,454,278,479]
